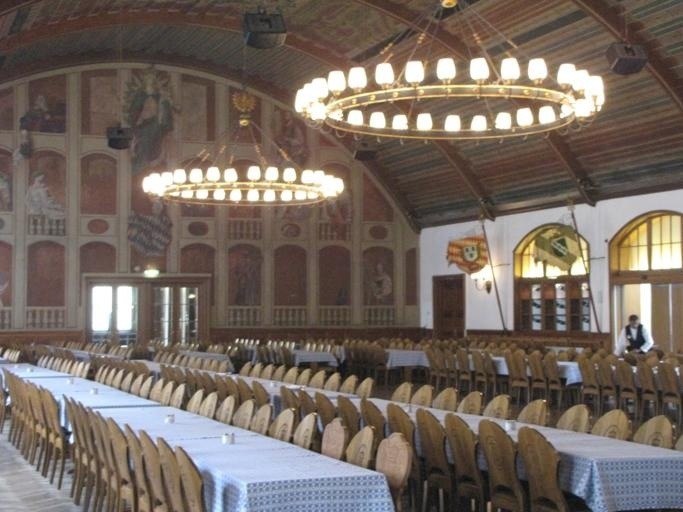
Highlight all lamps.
[141,35,344,207]
[602,8,648,77]
[242,6,288,49]
[292,0,610,140]
[470,262,497,293]
[104,126,135,152]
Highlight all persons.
[18,93,65,149]
[280,111,307,172]
[27,170,65,212]
[369,260,392,305]
[611,313,654,356]
[128,75,182,164]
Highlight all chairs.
[0,338,682,512]
